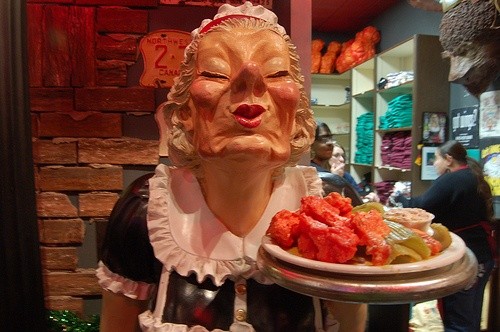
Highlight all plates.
[261,227,467,275]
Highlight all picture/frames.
[421,111,449,146]
[420,146,442,181]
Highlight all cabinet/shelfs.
[310,33,450,198]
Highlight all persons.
[309,122,360,193]
[329,142,345,170]
[96,2,367,332]
[395,139,495,332]
